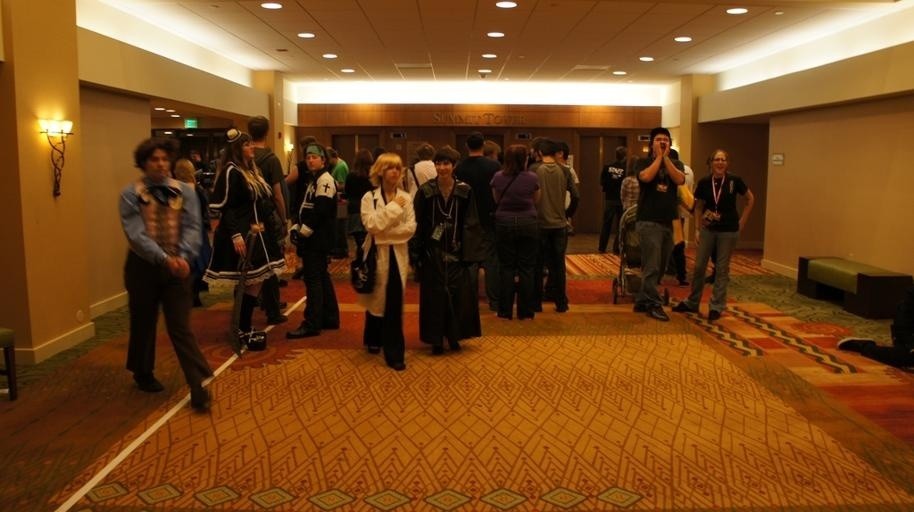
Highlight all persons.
[247,118,287,307]
[286,146,340,339]
[175,149,210,304]
[281,136,348,278]
[458,129,579,319]
[599,146,729,285]
[360,152,417,370]
[119,137,215,413]
[207,128,284,350]
[409,146,482,354]
[342,145,438,282]
[672,149,754,320]
[635,128,686,322]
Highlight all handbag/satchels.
[349,244,377,295]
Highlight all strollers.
[612,200,670,308]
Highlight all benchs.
[797,256,914,319]
[0,326,18,401]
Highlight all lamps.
[37,119,75,197]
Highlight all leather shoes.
[632,303,650,314]
[235,265,462,371]
[671,300,699,314]
[837,336,874,355]
[132,371,165,394]
[646,305,670,322]
[708,310,720,321]
